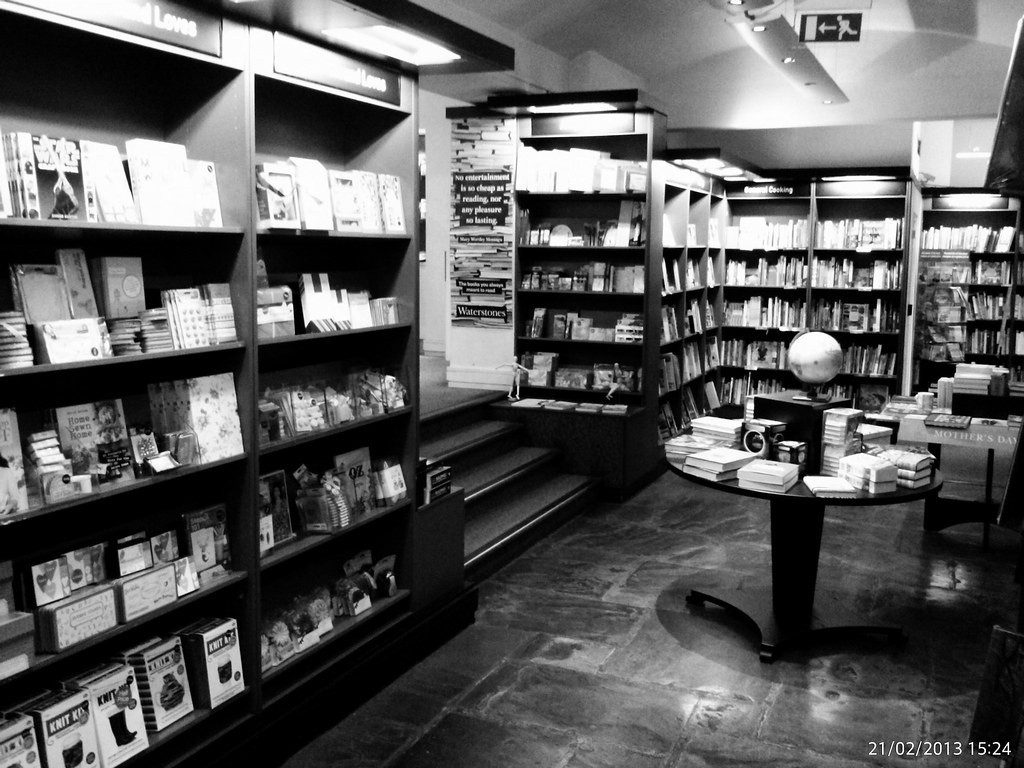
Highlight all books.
[662,217,1024,499]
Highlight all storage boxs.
[0,616,246,768]
[754,390,851,475]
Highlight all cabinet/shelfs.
[514,133,1024,471]
[0,0,420,768]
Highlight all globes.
[787,327,844,402]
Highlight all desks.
[422,483,465,659]
[878,396,1024,548]
[490,386,660,513]
[664,433,943,663]
